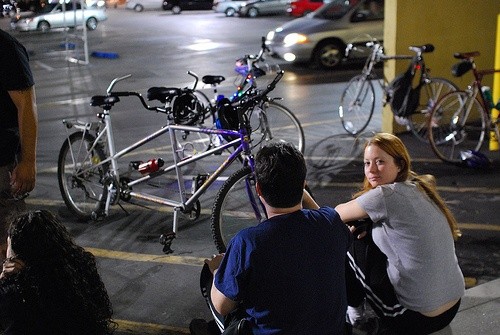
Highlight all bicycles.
[337,39,468,147]
[56,70,286,257]
[170,37,305,172]
[428,50,499,166]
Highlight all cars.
[125,0,320,18]
[261,0,384,71]
[10,1,108,33]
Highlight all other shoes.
[189,318,220,335]
[348,310,366,330]
[362,316,378,335]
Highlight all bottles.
[137,157,165,172]
[481,86,495,109]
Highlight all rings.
[3,257,16,263]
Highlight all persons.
[0,30,38,275]
[0,211,118,335]
[203,142,354,335]
[333,131,466,335]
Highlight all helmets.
[214,95,240,147]
[460,150,490,170]
[171,92,201,125]
[231,65,265,86]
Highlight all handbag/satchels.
[384,56,425,119]
